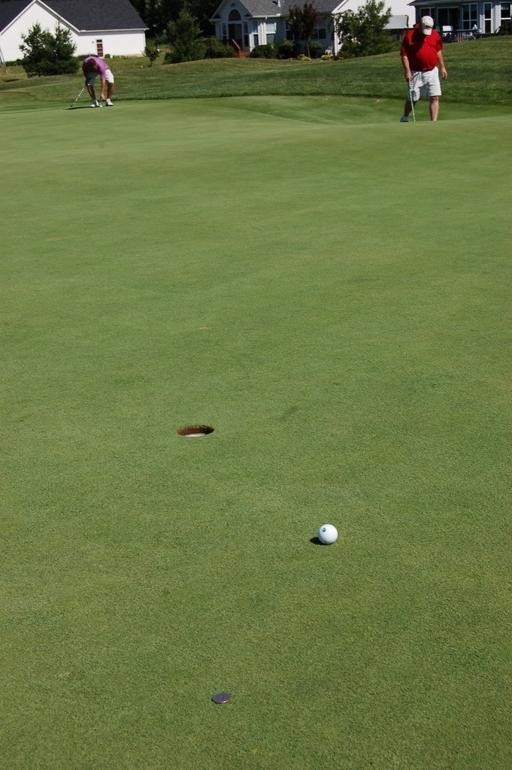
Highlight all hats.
[421,16,434,36]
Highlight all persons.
[82,55,115,108]
[399,15,449,123]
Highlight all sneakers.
[90,102,100,108]
[105,98,114,105]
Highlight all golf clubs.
[70,80,90,107]
[409,82,416,123]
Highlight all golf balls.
[99,105,102,107]
[319,524,337,544]
